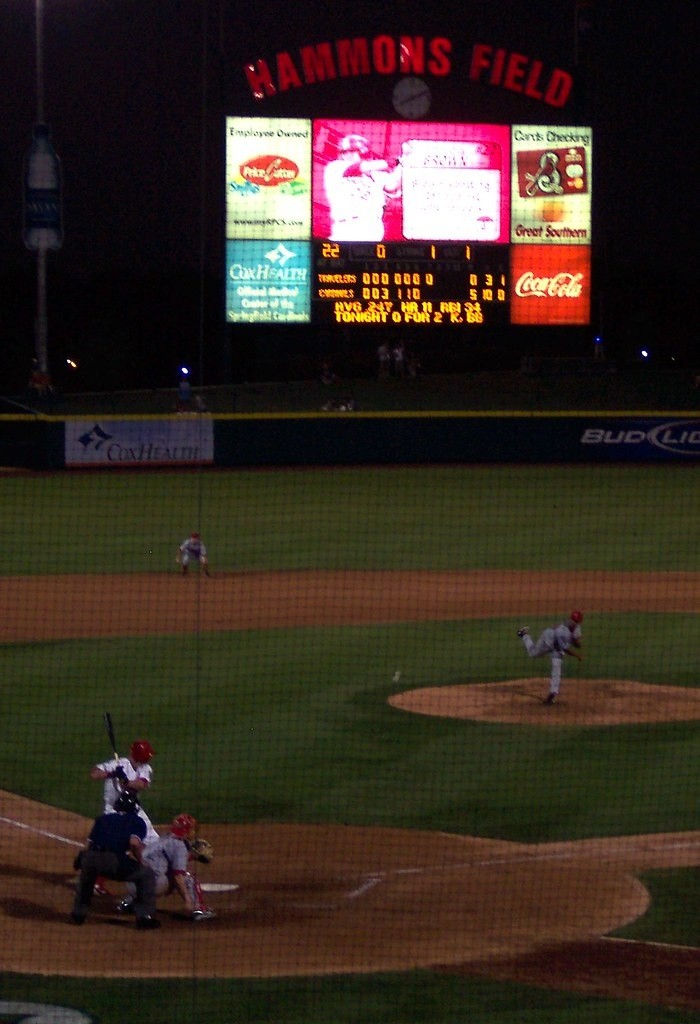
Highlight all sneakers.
[118,900,134,913]
[94,883,111,896]
[192,909,218,921]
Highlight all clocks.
[392,78,432,118]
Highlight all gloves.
[107,764,130,785]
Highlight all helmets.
[113,794,140,815]
[192,531,200,538]
[571,612,584,624]
[171,813,197,840]
[129,740,153,763]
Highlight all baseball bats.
[103,712,120,766]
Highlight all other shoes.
[136,915,150,929]
[71,911,86,925]
[517,627,529,637]
[547,693,556,705]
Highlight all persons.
[377,338,407,379]
[320,355,361,411]
[117,813,216,920]
[178,376,211,412]
[68,788,161,927]
[323,134,402,241]
[516,610,584,705]
[26,366,60,409]
[90,739,160,896]
[176,531,211,577]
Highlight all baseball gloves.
[193,839,214,863]
